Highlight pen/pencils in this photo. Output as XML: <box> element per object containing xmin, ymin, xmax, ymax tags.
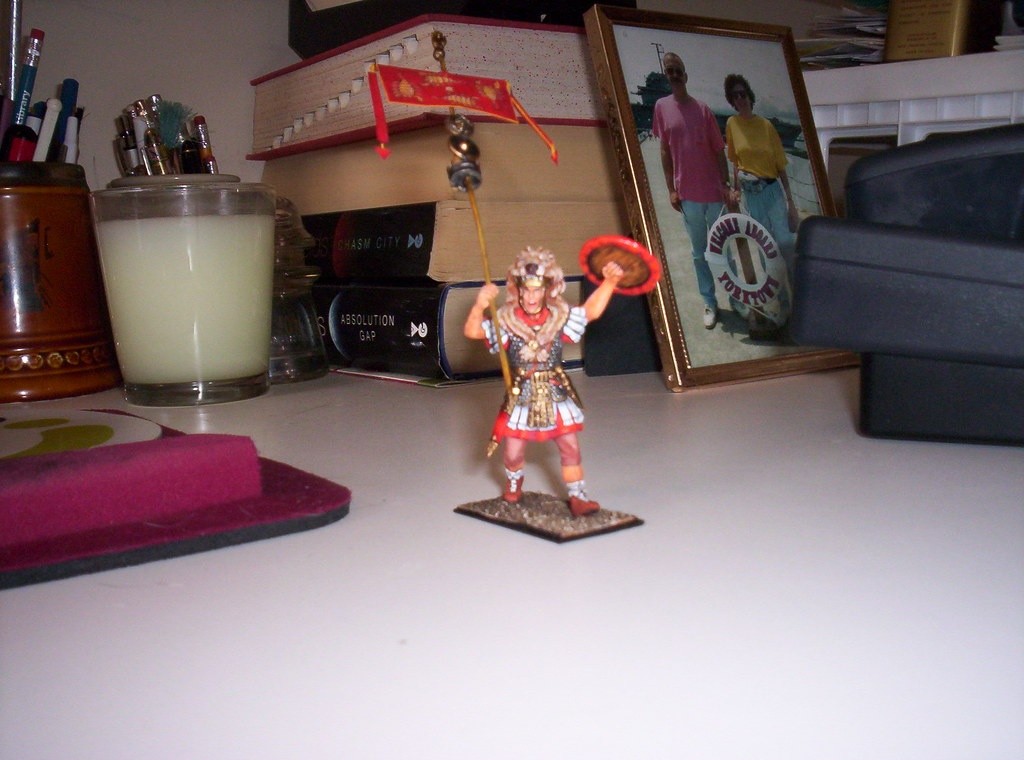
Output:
<box><xmin>115</xmin><ymin>94</ymin><xmax>219</xmax><ymax>176</ymax></box>
<box><xmin>12</xmin><ymin>27</ymin><xmax>44</xmax><ymax>123</ymax></box>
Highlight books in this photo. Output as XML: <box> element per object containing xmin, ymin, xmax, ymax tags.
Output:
<box><xmin>248</xmin><ymin>12</ymin><xmax>635</xmax><ymax>382</ymax></box>
<box><xmin>792</xmin><ymin>13</ymin><xmax>887</xmax><ymax>69</ymax></box>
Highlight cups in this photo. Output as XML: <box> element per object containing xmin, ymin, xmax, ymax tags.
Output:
<box><xmin>87</xmin><ymin>184</ymin><xmax>276</xmax><ymax>407</ymax></box>
<box><xmin>0</xmin><ymin>162</ymin><xmax>122</xmax><ymax>403</ymax></box>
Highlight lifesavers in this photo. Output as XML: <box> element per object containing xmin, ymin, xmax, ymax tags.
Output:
<box><xmin>703</xmin><ymin>211</ymin><xmax>787</xmax><ymax>308</ymax></box>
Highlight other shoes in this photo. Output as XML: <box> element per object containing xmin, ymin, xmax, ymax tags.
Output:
<box><xmin>703</xmin><ymin>295</ymin><xmax>719</xmax><ymax>329</ymax></box>
<box><xmin>731</xmin><ymin>299</ymin><xmax>751</xmax><ymax>320</ymax></box>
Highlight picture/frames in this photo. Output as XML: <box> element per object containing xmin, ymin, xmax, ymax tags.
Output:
<box><xmin>582</xmin><ymin>4</ymin><xmax>864</xmax><ymax>390</ymax></box>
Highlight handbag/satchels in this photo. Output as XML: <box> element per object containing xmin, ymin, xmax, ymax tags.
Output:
<box><xmin>738</xmin><ymin>170</ymin><xmax>768</xmax><ymax>194</ymax></box>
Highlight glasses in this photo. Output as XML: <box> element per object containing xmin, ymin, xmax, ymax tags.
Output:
<box><xmin>665</xmin><ymin>66</ymin><xmax>684</xmax><ymax>77</ymax></box>
<box><xmin>730</xmin><ymin>89</ymin><xmax>746</xmax><ymax>100</ymax></box>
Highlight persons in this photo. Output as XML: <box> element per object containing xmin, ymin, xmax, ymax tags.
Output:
<box><xmin>651</xmin><ymin>52</ymin><xmax>751</xmax><ymax>329</ymax></box>
<box><xmin>465</xmin><ymin>244</ymin><xmax>623</xmax><ymax>516</ymax></box>
<box><xmin>723</xmin><ymin>74</ymin><xmax>800</xmax><ymax>320</ymax></box>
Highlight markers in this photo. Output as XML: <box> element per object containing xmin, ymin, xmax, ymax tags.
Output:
<box><xmin>0</xmin><ymin>78</ymin><xmax>83</xmax><ymax>163</ymax></box>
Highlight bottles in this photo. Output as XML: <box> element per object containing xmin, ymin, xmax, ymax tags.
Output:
<box><xmin>268</xmin><ymin>196</ymin><xmax>329</xmax><ymax>383</ymax></box>
